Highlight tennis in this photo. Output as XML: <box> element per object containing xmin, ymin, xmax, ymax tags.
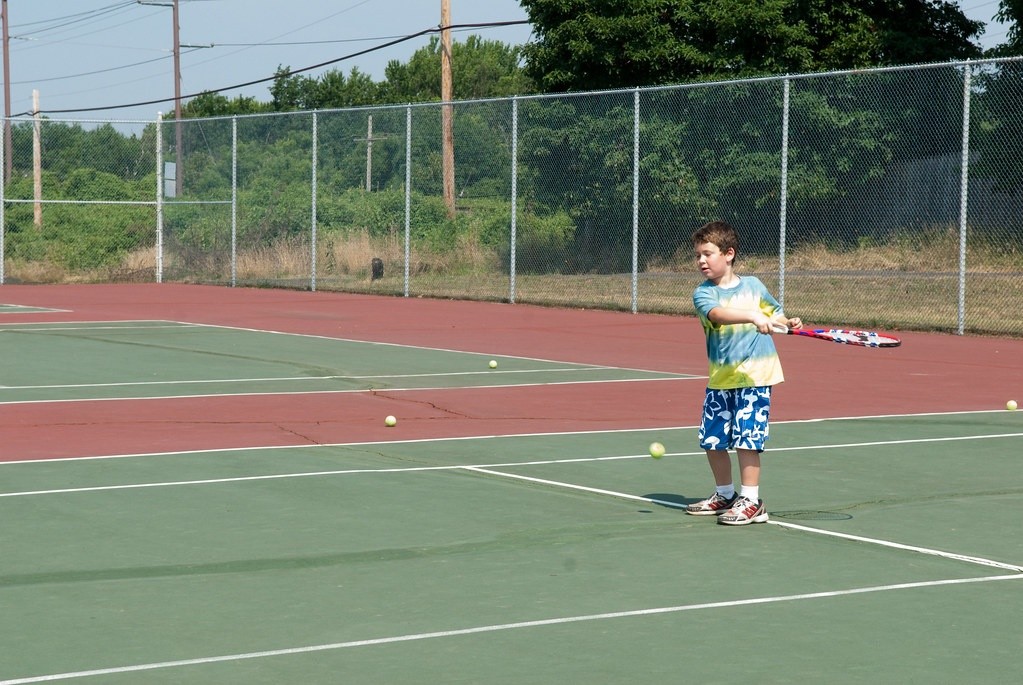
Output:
<box><xmin>649</xmin><ymin>441</ymin><xmax>665</xmax><ymax>458</ymax></box>
<box><xmin>385</xmin><ymin>415</ymin><xmax>397</xmax><ymax>427</ymax></box>
<box><xmin>1006</xmin><ymin>400</ymin><xmax>1019</xmax><ymax>411</ymax></box>
<box><xmin>489</xmin><ymin>359</ymin><xmax>498</xmax><ymax>369</ymax></box>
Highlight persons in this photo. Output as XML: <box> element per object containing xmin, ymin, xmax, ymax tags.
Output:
<box><xmin>686</xmin><ymin>222</ymin><xmax>803</xmax><ymax>526</ymax></box>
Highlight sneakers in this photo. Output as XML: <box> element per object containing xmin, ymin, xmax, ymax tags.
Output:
<box><xmin>716</xmin><ymin>497</ymin><xmax>769</xmax><ymax>524</ymax></box>
<box><xmin>687</xmin><ymin>490</ymin><xmax>739</xmax><ymax>515</ymax></box>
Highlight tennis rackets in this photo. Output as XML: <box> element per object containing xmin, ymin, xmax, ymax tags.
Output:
<box><xmin>755</xmin><ymin>321</ymin><xmax>902</xmax><ymax>348</ymax></box>
